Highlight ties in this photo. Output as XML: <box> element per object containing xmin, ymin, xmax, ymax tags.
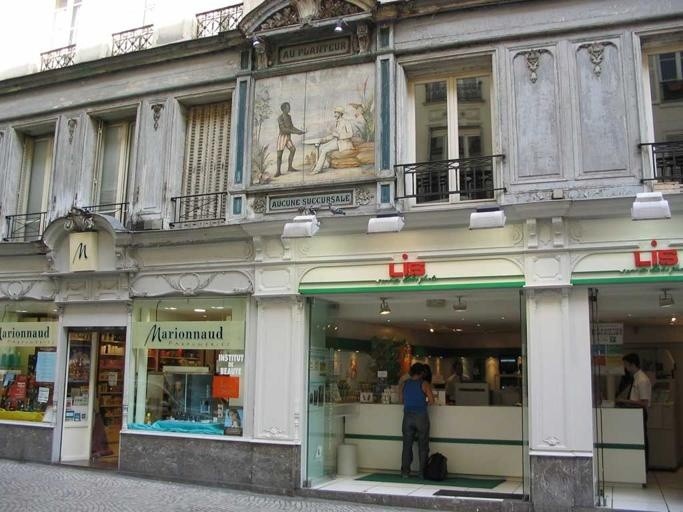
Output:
<box><xmin>626</xmin><ymin>376</ymin><xmax>634</xmax><ymax>400</ymax></box>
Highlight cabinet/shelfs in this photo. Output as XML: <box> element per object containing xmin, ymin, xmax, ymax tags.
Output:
<box><xmin>68</xmin><ymin>333</ymin><xmax>206</xmax><ymax>445</ymax></box>
<box><xmin>646</xmin><ymin>379</ymin><xmax>683</xmax><ymax>473</ymax></box>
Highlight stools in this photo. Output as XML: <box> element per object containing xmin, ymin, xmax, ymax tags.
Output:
<box><xmin>327</xmin><ymin>148</ymin><xmax>361</xmax><ymax>168</ymax></box>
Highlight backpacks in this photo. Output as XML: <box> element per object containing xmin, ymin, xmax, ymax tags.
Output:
<box><xmin>423</xmin><ymin>452</ymin><xmax>448</xmax><ymax>481</ymax></box>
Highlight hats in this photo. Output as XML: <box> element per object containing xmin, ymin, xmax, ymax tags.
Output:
<box><xmin>333</xmin><ymin>106</ymin><xmax>347</xmax><ymax>114</ymax></box>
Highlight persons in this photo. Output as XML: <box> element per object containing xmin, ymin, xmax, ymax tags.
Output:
<box><xmin>400</xmin><ymin>363</ymin><xmax>434</xmax><ymax>478</ymax></box>
<box><xmin>274</xmin><ymin>102</ymin><xmax>305</xmax><ymax>177</ymax></box>
<box><xmin>398</xmin><ymin>364</ymin><xmax>432</xmax><ymax>383</ymax></box>
<box><xmin>614</xmin><ymin>353</ymin><xmax>652</xmax><ymax>488</ymax></box>
<box><xmin>309</xmin><ymin>106</ymin><xmax>353</xmax><ymax>175</ymax></box>
<box><xmin>228</xmin><ymin>409</ymin><xmax>241</xmax><ymax>432</ymax></box>
<box><xmin>446</xmin><ymin>362</ymin><xmax>469</xmax><ymax>404</ymax></box>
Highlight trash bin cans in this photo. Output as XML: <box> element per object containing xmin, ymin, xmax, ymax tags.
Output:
<box><xmin>336</xmin><ymin>443</ymin><xmax>357</xmax><ymax>476</ymax></box>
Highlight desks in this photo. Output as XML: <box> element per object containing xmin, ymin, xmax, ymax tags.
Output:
<box><xmin>302</xmin><ymin>138</ymin><xmax>329</xmax><ymax>172</ymax></box>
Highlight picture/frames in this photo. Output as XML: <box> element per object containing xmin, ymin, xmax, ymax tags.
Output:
<box><xmin>33</xmin><ymin>346</ymin><xmax>57</xmax><ymax>386</ymax></box>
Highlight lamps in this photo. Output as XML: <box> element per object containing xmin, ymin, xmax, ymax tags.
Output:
<box><xmin>283</xmin><ymin>206</ymin><xmax>320</xmax><ymax>237</ymax></box>
<box><xmin>251</xmin><ymin>32</ymin><xmax>264</xmax><ymax>48</ymax></box>
<box><xmin>658</xmin><ymin>288</ymin><xmax>676</xmax><ymax>308</ymax></box>
<box><xmin>452</xmin><ymin>295</ymin><xmax>467</xmax><ymax>312</ymax></box>
<box><xmin>631</xmin><ymin>180</ymin><xmax>672</xmax><ymax>220</ymax></box>
<box><xmin>333</xmin><ymin>18</ymin><xmax>356</xmax><ymax>35</ymax></box>
<box><xmin>367</xmin><ymin>212</ymin><xmax>405</xmax><ymax>233</ymax></box>
<box><xmin>377</xmin><ymin>296</ymin><xmax>391</xmax><ymax>315</ymax></box>
<box><xmin>468</xmin><ymin>206</ymin><xmax>507</xmax><ymax>230</ymax></box>
<box><xmin>63</xmin><ymin>206</ymin><xmax>94</xmax><ymax>232</ymax></box>
